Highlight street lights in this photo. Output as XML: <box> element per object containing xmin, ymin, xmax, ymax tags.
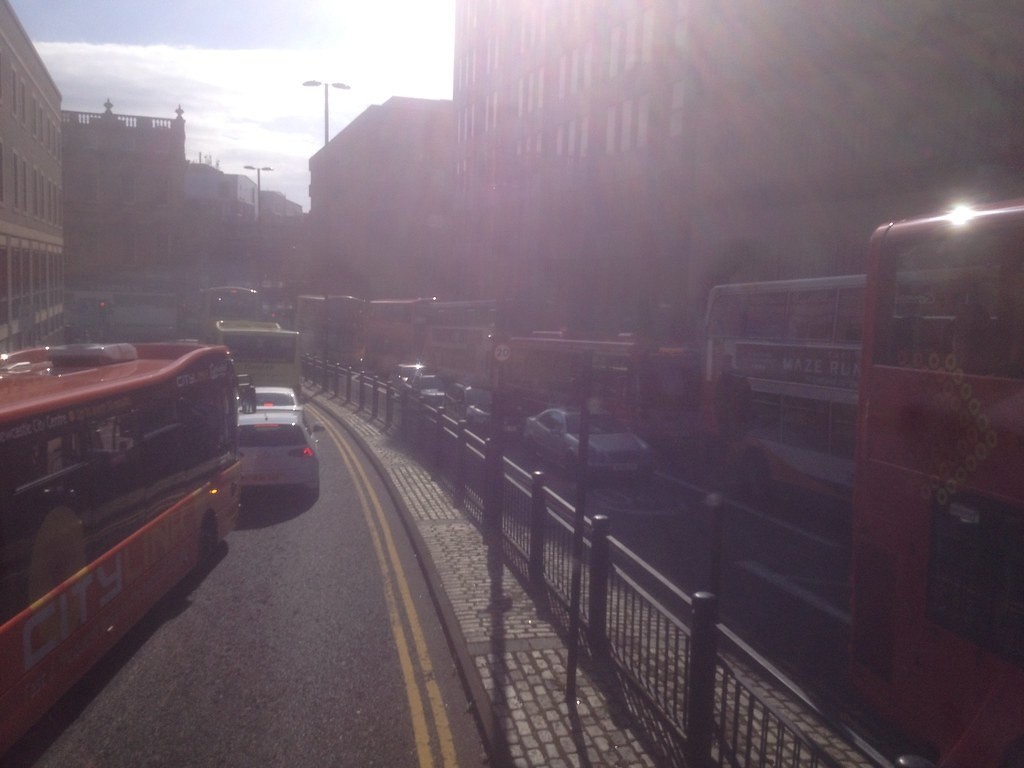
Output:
<box><xmin>244</xmin><ymin>164</ymin><xmax>275</xmax><ymax>220</ymax></box>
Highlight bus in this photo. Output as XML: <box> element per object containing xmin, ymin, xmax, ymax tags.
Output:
<box><xmin>705</xmin><ymin>272</ymin><xmax>941</xmax><ymax>412</ymax></box>
<box><xmin>216</xmin><ymin>318</ymin><xmax>302</xmax><ymax>397</ymax></box>
<box><xmin>0</xmin><ymin>338</ymin><xmax>258</xmax><ymax>755</ymax></box>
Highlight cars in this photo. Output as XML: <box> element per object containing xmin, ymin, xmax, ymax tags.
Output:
<box><xmin>525</xmin><ymin>406</ymin><xmax>654</xmax><ymax>479</ymax></box>
<box><xmin>236</xmin><ymin>386</ymin><xmax>313</xmax><ymax>439</ymax></box>
<box><xmin>236</xmin><ymin>410</ymin><xmax>326</xmax><ymax>497</ymax></box>
<box><xmin>393</xmin><ymin>356</ymin><xmax>531</xmax><ymax>443</ymax></box>
